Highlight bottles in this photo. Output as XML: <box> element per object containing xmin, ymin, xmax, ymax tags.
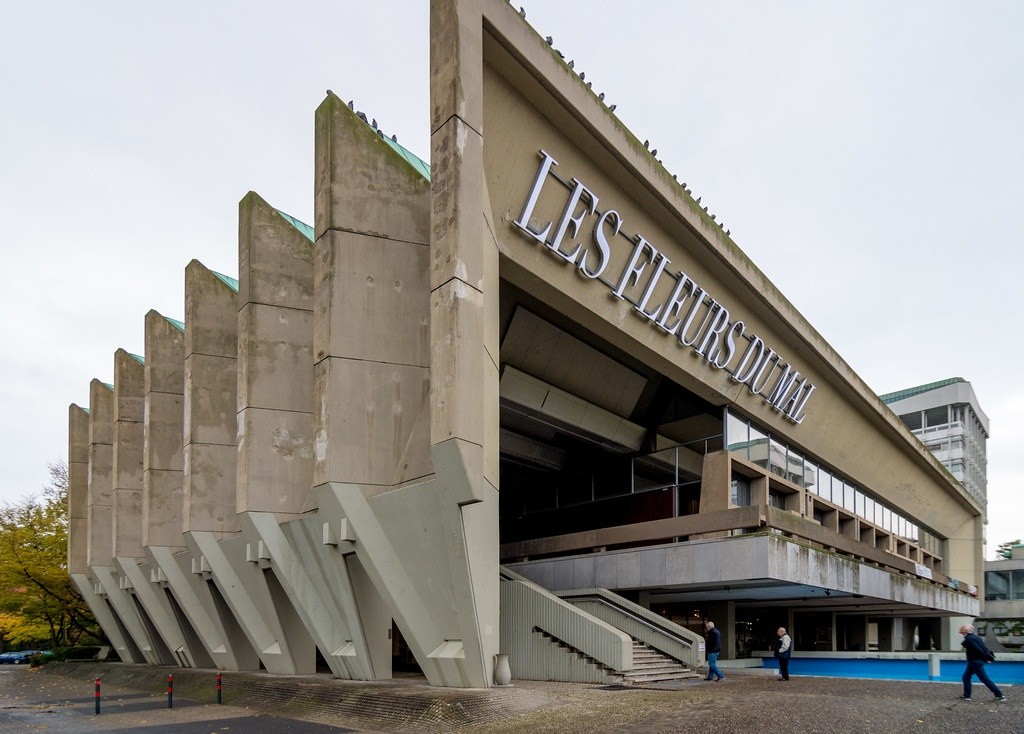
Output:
<box><xmin>495</xmin><ymin>654</ymin><xmax>511</xmax><ymax>685</ymax></box>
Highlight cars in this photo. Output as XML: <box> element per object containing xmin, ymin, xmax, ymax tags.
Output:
<box><xmin>0</xmin><ymin>649</ymin><xmax>44</xmax><ymax>665</ymax></box>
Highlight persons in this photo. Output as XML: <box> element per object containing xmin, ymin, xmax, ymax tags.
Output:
<box><xmin>959</xmin><ymin>625</ymin><xmax>1008</xmax><ymax>702</ymax></box>
<box><xmin>704</xmin><ymin>621</ymin><xmax>725</xmax><ymax>681</ymax></box>
<box><xmin>774</xmin><ymin>627</ymin><xmax>792</xmax><ymax>681</ymax></box>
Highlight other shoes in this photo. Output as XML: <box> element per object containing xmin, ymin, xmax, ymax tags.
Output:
<box><xmin>778</xmin><ymin>678</ymin><xmax>788</xmax><ymax>681</ymax></box>
<box><xmin>959</xmin><ymin>696</ymin><xmax>972</xmax><ymax>701</ymax></box>
<box><xmin>715</xmin><ymin>677</ymin><xmax>725</xmax><ymax>682</ymax></box>
<box><xmin>704</xmin><ymin>677</ymin><xmax>713</xmax><ymax>682</ymax></box>
<box><xmin>993</xmin><ymin>695</ymin><xmax>1007</xmax><ymax>703</ymax></box>
